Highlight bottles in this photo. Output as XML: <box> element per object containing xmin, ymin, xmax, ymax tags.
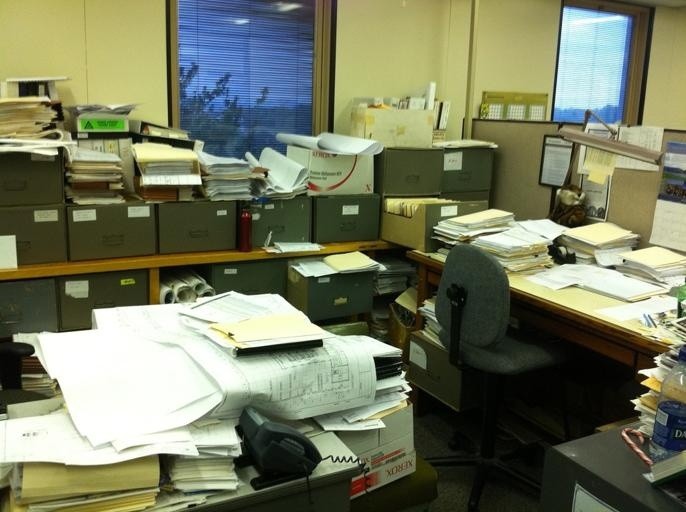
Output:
<box><xmin>648</xmin><ymin>345</ymin><xmax>686</xmax><ymax>465</ymax></box>
<box><xmin>237</xmin><ymin>205</ymin><xmax>253</xmax><ymax>253</ymax></box>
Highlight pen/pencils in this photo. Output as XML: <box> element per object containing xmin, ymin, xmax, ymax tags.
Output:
<box><xmin>644</xmin><ymin>313</ymin><xmax>657</xmax><ymax>328</ymax></box>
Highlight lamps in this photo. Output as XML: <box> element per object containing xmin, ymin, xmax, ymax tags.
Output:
<box><xmin>548</xmin><ymin>107</ymin><xmax>669</xmax><ymax>229</ymax></box>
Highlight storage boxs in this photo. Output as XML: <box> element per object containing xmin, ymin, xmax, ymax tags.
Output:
<box><xmin>0</xmin><ymin>138</ymin><xmax>495</xmax><ymax>414</ymax></box>
<box><xmin>348</xmin><ymin>95</ymin><xmax>435</xmax><ymax>145</ymax></box>
<box><xmin>538</xmin><ymin>420</ymin><xmax>685</xmax><ymax>511</ymax></box>
<box><xmin>336</xmin><ymin>400</ymin><xmax>419</xmax><ymax>503</ymax></box>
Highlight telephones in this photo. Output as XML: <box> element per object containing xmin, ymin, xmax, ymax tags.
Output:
<box><xmin>235</xmin><ymin>405</ymin><xmax>322</xmax><ymax>475</ymax></box>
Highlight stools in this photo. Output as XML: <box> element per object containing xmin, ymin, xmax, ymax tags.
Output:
<box><xmin>353</xmin><ymin>450</ymin><xmax>439</xmax><ymax>511</ymax></box>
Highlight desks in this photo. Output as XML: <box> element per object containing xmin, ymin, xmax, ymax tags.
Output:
<box><xmin>169</xmin><ymin>430</ymin><xmax>361</xmax><ymax>511</ymax></box>
<box><xmin>402</xmin><ymin>248</ymin><xmax>673</xmax><ymax>415</ymax></box>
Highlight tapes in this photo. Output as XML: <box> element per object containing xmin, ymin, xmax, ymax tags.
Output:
<box><xmin>558</xmin><ymin>246</ymin><xmax>568</xmax><ymax>256</ymax></box>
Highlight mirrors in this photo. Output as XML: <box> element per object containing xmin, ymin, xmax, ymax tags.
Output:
<box><xmin>463</xmin><ymin>0</ymin><xmax>685</xmax><ymax>207</ymax></box>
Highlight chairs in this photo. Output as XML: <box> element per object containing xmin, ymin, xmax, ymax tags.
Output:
<box><xmin>435</xmin><ymin>242</ymin><xmax>581</xmax><ymax>512</ymax></box>
